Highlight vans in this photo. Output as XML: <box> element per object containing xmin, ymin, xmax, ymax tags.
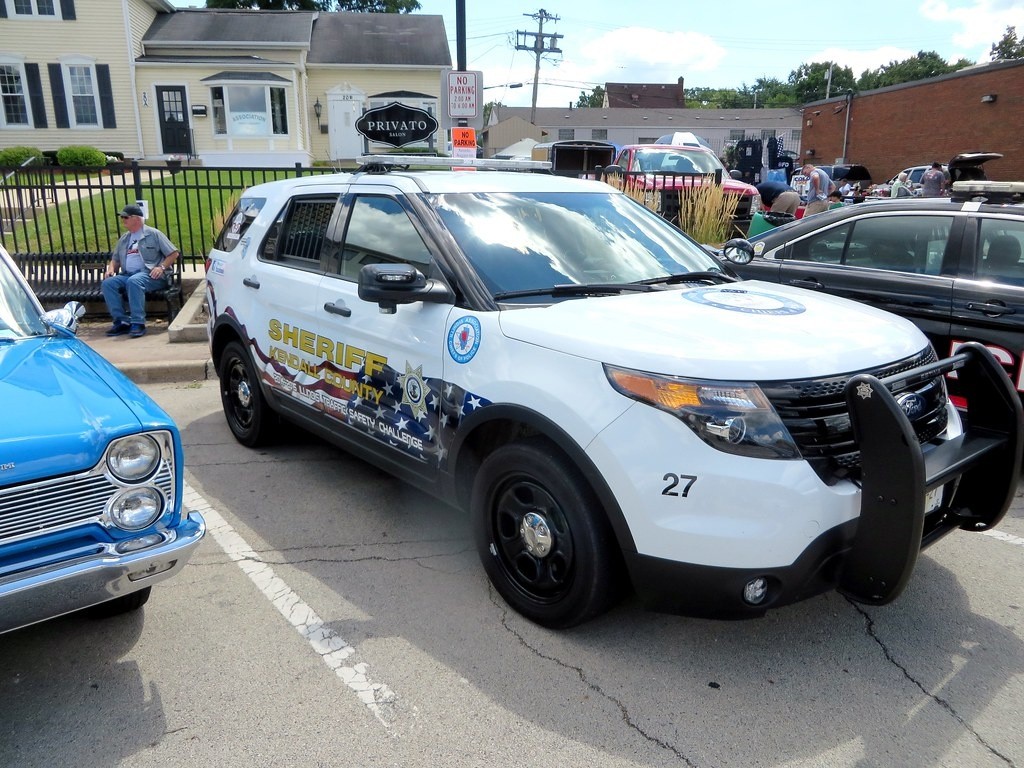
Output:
<box><xmin>791</xmin><ymin>164</ymin><xmax>871</xmax><ymax>195</ymax></box>
<box><xmin>883</xmin><ymin>152</ymin><xmax>1004</xmax><ymax>200</ymax></box>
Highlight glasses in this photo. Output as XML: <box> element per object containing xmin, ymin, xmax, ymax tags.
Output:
<box><xmin>121</xmin><ymin>216</ymin><xmax>134</xmax><ymax>219</ymax></box>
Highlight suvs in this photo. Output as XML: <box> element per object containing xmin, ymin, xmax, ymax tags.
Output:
<box><xmin>205</xmin><ymin>153</ymin><xmax>1024</xmax><ymax>631</ymax></box>
<box><xmin>607</xmin><ymin>144</ymin><xmax>761</xmax><ymax>241</ymax></box>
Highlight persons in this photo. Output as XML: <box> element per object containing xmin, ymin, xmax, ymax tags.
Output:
<box><xmin>756</xmin><ymin>181</ymin><xmax>800</xmax><ymax>217</ymax></box>
<box><xmin>100</xmin><ymin>205</ymin><xmax>180</xmax><ymax>338</ymax></box>
<box><xmin>891</xmin><ymin>173</ymin><xmax>915</xmax><ymax>199</ymax></box>
<box><xmin>829</xmin><ymin>190</ymin><xmax>845</xmax><ymax>209</ymax></box>
<box><xmin>919</xmin><ymin>161</ymin><xmax>946</xmax><ymax>197</ymax></box>
<box><xmin>838</xmin><ymin>179</ymin><xmax>852</xmax><ymax>195</ymax></box>
<box><xmin>801</xmin><ymin>163</ymin><xmax>836</xmax><ymax>219</ymax></box>
<box><xmin>938</xmin><ymin>164</ymin><xmax>951</xmax><ymax>185</ymax></box>
<box><xmin>848</xmin><ymin>182</ymin><xmax>862</xmax><ymax>197</ymax></box>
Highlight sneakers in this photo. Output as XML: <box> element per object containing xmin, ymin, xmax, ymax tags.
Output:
<box><xmin>105</xmin><ymin>324</ymin><xmax>132</xmax><ymax>335</ymax></box>
<box><xmin>129</xmin><ymin>323</ymin><xmax>146</xmax><ymax>336</ymax></box>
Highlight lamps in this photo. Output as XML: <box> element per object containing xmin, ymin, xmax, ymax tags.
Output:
<box><xmin>980</xmin><ymin>94</ymin><xmax>997</xmax><ymax>102</ymax></box>
<box><xmin>314</xmin><ymin>97</ymin><xmax>322</xmax><ymax>129</ymax></box>
<box><xmin>806</xmin><ymin>149</ymin><xmax>816</xmax><ymax>155</ymax></box>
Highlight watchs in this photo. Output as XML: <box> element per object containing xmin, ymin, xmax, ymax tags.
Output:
<box><xmin>159</xmin><ymin>263</ymin><xmax>166</xmax><ymax>270</ymax></box>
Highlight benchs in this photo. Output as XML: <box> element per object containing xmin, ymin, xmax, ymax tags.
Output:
<box><xmin>10</xmin><ymin>250</ymin><xmax>185</xmax><ymax>328</ymax></box>
<box><xmin>833</xmin><ymin>240</ymin><xmax>947</xmax><ymax>274</ymax></box>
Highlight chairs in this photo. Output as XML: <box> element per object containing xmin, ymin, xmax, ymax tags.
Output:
<box><xmin>985</xmin><ymin>236</ymin><xmax>1024</xmax><ymax>289</ymax></box>
<box><xmin>676</xmin><ymin>157</ymin><xmax>696</xmax><ymax>173</ymax></box>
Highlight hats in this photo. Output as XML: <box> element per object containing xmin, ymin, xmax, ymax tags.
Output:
<box><xmin>115</xmin><ymin>205</ymin><xmax>143</xmax><ymax>217</ymax></box>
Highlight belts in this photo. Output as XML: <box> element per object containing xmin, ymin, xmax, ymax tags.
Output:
<box><xmin>782</xmin><ymin>190</ymin><xmax>797</xmax><ymax>192</ymax></box>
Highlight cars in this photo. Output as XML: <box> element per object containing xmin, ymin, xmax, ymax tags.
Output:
<box><xmin>0</xmin><ymin>244</ymin><xmax>205</xmax><ymax>638</ymax></box>
<box><xmin>705</xmin><ymin>180</ymin><xmax>1024</xmax><ymax>434</ymax></box>
